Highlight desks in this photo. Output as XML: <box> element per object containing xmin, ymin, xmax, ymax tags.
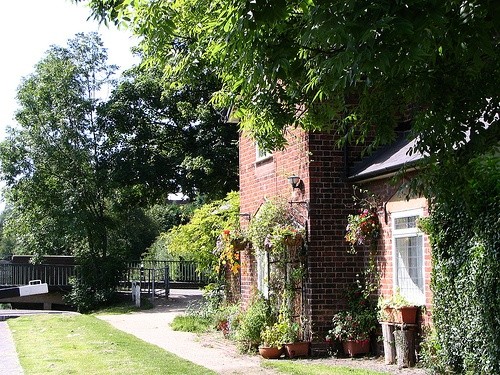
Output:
<box><xmin>379</xmin><ymin>322</ymin><xmax>420</xmax><ymax>369</ymax></box>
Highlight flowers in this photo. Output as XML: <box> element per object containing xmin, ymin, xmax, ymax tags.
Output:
<box><xmin>324</xmin><ymin>285</ymin><xmax>378</xmax><ymax>356</ymax></box>
<box><xmin>342</xmin><ymin>183</ymin><xmax>380</xmax><ymax>254</ymax></box>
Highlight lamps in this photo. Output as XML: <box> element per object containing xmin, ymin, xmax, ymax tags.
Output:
<box><xmin>288</xmin><ymin>173</ymin><xmax>301</xmax><ymax>189</ymax></box>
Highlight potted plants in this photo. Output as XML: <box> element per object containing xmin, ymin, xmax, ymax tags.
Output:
<box><xmin>376</xmin><ymin>290</ymin><xmax>417</xmax><ymax>324</ymax></box>
<box><xmin>214</xmin><ymin>199</ymin><xmax>309</xmax><ymax>358</ymax></box>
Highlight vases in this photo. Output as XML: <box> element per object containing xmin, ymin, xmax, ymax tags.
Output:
<box><xmin>342</xmin><ymin>339</ymin><xmax>369</xmax><ymax>355</ymax></box>
